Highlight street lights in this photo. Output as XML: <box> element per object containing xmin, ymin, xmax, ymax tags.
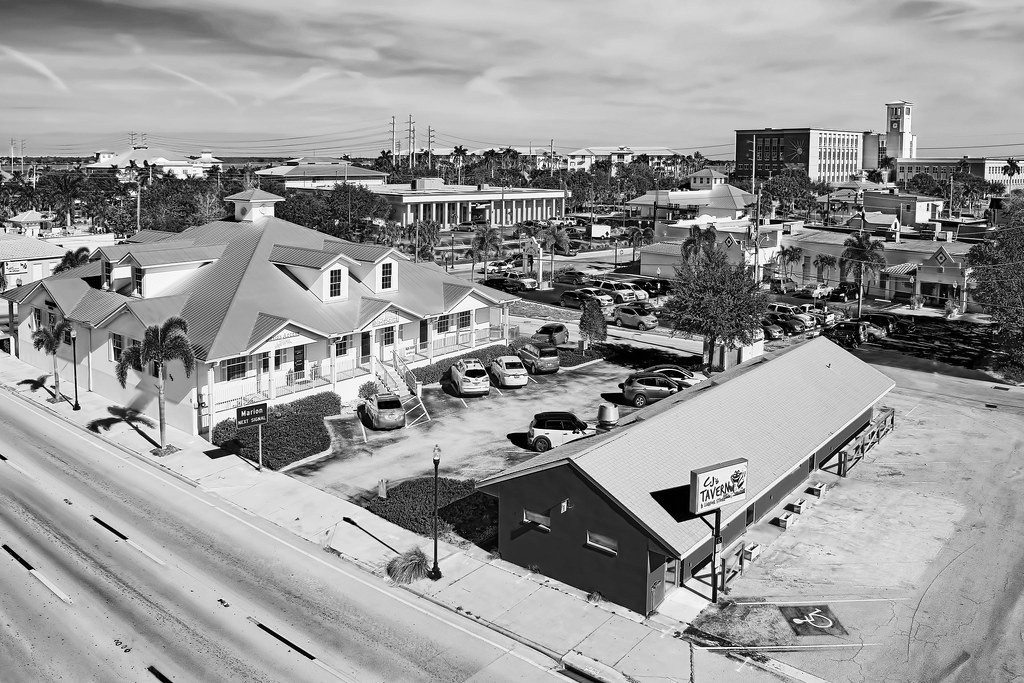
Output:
<box><xmin>429</xmin><ymin>444</ymin><xmax>442</xmax><ymax>580</ymax></box>
<box><xmin>70</xmin><ymin>329</ymin><xmax>81</xmax><ymax>410</ymax></box>
<box><xmin>754</xmin><ymin>234</ymin><xmax>770</xmax><ymax>284</ymax></box>
<box><xmin>656</xmin><ymin>267</ymin><xmax>661</xmax><ymax>304</ymax></box>
<box><xmin>909</xmin><ymin>276</ymin><xmax>914</xmax><ymax>309</ymax></box>
<box><xmin>614</xmin><ymin>239</ymin><xmax>618</xmax><ymax>269</ymax></box>
<box><xmin>451</xmin><ymin>233</ymin><xmax>454</xmax><ymax>269</ymax></box>
<box><xmin>953</xmin><ymin>280</ymin><xmax>958</xmax><ymax>304</ymax></box>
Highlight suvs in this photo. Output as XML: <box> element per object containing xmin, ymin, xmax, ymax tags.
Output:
<box><xmin>770</xmin><ymin>277</ymin><xmax>798</xmax><ymax>295</ymax></box>
<box><xmin>830</xmin><ymin>281</ymin><xmax>860</xmax><ymax>302</ymax></box>
<box><xmin>623</xmin><ymin>371</ymin><xmax>692</xmax><ymax>408</ymax></box>
<box><xmin>450</xmin><ymin>357</ymin><xmax>491</xmax><ymax>396</ymax></box>
<box><xmin>451</xmin><ymin>220</ymin><xmax>490</xmax><ymax>232</ymax></box>
<box><xmin>518</xmin><ymin>343</ymin><xmax>560</xmax><ymax>375</ymax></box>
<box><xmin>526</xmin><ymin>411</ymin><xmax>596</xmax><ymax>453</ymax></box>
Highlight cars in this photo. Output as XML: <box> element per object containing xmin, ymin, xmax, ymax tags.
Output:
<box><xmin>365</xmin><ymin>393</ymin><xmax>405</xmax><ymax>430</ymax></box>
<box><xmin>479</xmin><ymin>253</ymin><xmax>538</xmax><ymax>293</ymax></box>
<box><xmin>513</xmin><ymin>216</ymin><xmax>611</xmax><ymax>256</ymax></box>
<box><xmin>561</xmin><ymin>279</ymin><xmax>679</xmax><ymax>331</ymax></box>
<box><xmin>750</xmin><ymin>302</ymin><xmax>916</xmax><ymax>350</ymax></box>
<box><xmin>801</xmin><ymin>282</ymin><xmax>835</xmax><ymax>300</ymax></box>
<box><xmin>636</xmin><ymin>364</ymin><xmax>709</xmax><ymax>386</ymax></box>
<box><xmin>491</xmin><ymin>355</ymin><xmax>529</xmax><ymax>388</ymax></box>
<box><xmin>531</xmin><ymin>323</ymin><xmax>569</xmax><ymax>345</ymax></box>
<box><xmin>554</xmin><ymin>271</ymin><xmax>590</xmax><ymax>286</ymax></box>
